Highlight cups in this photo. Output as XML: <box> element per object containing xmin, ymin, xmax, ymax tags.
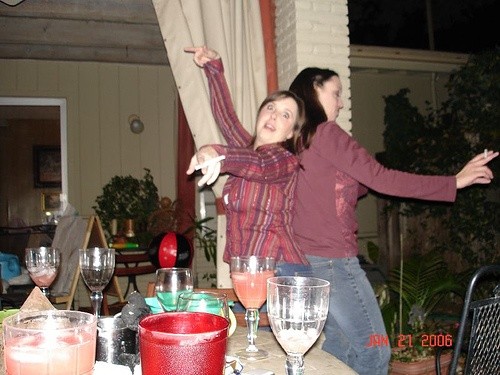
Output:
<box><xmin>178</xmin><ymin>292</ymin><xmax>229</xmax><ymax>320</ymax></box>
<box><xmin>95</xmin><ymin>317</ymin><xmax>136</xmax><ymax>374</ymax></box>
<box><xmin>155</xmin><ymin>267</ymin><xmax>193</xmax><ymax>312</ymax></box>
<box><xmin>2</xmin><ymin>309</ymin><xmax>97</xmax><ymax>375</ymax></box>
<box><xmin>138</xmin><ymin>311</ymin><xmax>229</xmax><ymax>375</ymax></box>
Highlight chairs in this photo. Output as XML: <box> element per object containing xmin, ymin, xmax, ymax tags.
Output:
<box><xmin>435</xmin><ymin>262</ymin><xmax>500</xmax><ymax>375</ymax></box>
<box><xmin>114</xmin><ymin>250</ymin><xmax>160</xmax><ymax>278</ymax></box>
<box><xmin>145</xmin><ymin>281</ymin><xmax>271</xmax><ymax>327</ymax></box>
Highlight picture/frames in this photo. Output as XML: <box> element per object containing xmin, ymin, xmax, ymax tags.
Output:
<box><xmin>32</xmin><ymin>144</ymin><xmax>61</xmax><ymax>189</ymax></box>
<box><xmin>40</xmin><ymin>191</ymin><xmax>61</xmax><ymax>212</ymax></box>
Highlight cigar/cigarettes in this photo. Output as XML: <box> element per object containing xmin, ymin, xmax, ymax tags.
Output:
<box><xmin>483</xmin><ymin>147</ymin><xmax>487</xmax><ymax>160</ymax></box>
<box><xmin>195</xmin><ymin>154</ymin><xmax>226</xmax><ymax>171</ymax></box>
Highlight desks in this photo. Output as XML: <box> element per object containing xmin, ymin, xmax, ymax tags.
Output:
<box><xmin>224</xmin><ymin>324</ymin><xmax>360</xmax><ymax>375</ymax></box>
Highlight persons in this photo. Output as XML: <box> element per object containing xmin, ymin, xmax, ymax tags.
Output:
<box><xmin>185</xmin><ymin>45</ymin><xmax>314</xmax><ymax>311</ymax></box>
<box><xmin>287</xmin><ymin>66</ymin><xmax>499</xmax><ymax>375</ymax></box>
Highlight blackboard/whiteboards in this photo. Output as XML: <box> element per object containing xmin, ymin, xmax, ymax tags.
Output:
<box><xmin>45</xmin><ymin>214</ymin><xmax>121</xmax><ymax>303</ymax></box>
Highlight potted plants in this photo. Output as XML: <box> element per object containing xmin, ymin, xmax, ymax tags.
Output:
<box><xmin>355</xmin><ymin>234</ymin><xmax>486</xmax><ymax>375</ymax></box>
<box><xmin>89</xmin><ymin>166</ymin><xmax>161</xmax><ymax>245</ymax></box>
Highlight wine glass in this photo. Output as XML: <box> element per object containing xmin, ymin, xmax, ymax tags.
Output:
<box><xmin>267</xmin><ymin>276</ymin><xmax>330</xmax><ymax>375</ymax></box>
<box><xmin>24</xmin><ymin>248</ymin><xmax>60</xmax><ymax>299</ymax></box>
<box><xmin>230</xmin><ymin>256</ymin><xmax>277</xmax><ymax>360</ymax></box>
<box><xmin>78</xmin><ymin>248</ymin><xmax>116</xmax><ymax>318</ymax></box>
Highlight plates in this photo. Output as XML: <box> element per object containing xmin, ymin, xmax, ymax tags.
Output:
<box><xmin>224</xmin><ymin>355</ymin><xmax>243</xmax><ymax>375</ymax></box>
<box><xmin>146</xmin><ymin>296</ymin><xmax>238</xmax><ymax>338</ymax></box>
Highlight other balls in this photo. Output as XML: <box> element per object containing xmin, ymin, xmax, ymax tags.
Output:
<box><xmin>147</xmin><ymin>231</ymin><xmax>193</xmax><ymax>273</ymax></box>
